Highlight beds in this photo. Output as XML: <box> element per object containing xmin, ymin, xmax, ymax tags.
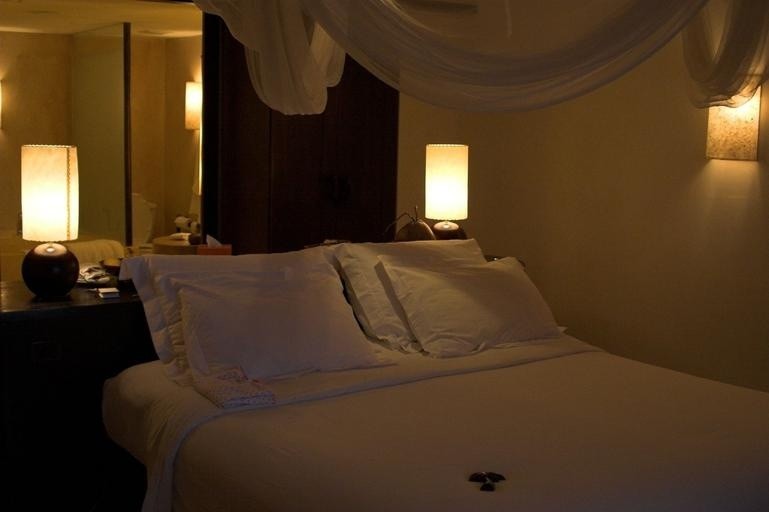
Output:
<box><xmin>101</xmin><ymin>335</ymin><xmax>768</xmax><ymax>512</ymax></box>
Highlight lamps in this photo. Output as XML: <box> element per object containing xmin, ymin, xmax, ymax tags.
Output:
<box><xmin>22</xmin><ymin>144</ymin><xmax>80</xmax><ymax>302</ymax></box>
<box><xmin>424</xmin><ymin>144</ymin><xmax>469</xmax><ymax>240</ymax></box>
<box><xmin>705</xmin><ymin>74</ymin><xmax>763</xmax><ymax>162</ymax></box>
<box><xmin>185</xmin><ymin>82</ymin><xmax>200</xmax><ymax>130</ymax></box>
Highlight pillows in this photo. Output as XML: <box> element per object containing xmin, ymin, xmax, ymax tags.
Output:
<box><xmin>329</xmin><ymin>236</ymin><xmax>487</xmax><ymax>352</ymax></box>
<box><xmin>379</xmin><ymin>252</ymin><xmax>568</xmax><ymax>358</ymax></box>
<box><xmin>156</xmin><ymin>264</ymin><xmax>399</xmax><ymax>382</ymax></box>
<box><xmin>117</xmin><ymin>244</ymin><xmax>343</xmax><ymax>387</ymax></box>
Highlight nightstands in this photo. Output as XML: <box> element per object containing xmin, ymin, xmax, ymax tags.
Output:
<box><xmin>0</xmin><ymin>274</ymin><xmax>139</xmax><ymax>326</ymax></box>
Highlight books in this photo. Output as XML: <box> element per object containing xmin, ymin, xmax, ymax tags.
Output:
<box><xmin>98</xmin><ymin>287</ymin><xmax>119</xmax><ymax>299</ymax></box>
